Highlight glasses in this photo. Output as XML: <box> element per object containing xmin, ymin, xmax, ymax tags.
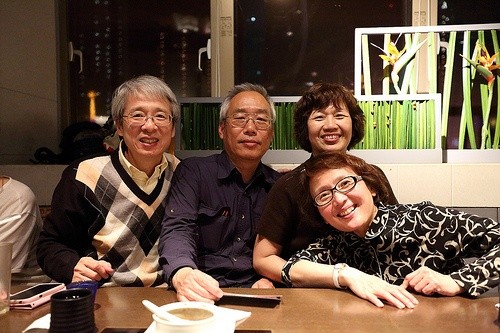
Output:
<box><xmin>226</xmin><ymin>112</ymin><xmax>274</xmax><ymax>130</ymax></box>
<box><xmin>313</xmin><ymin>175</ymin><xmax>362</xmax><ymax>206</ymax></box>
<box><xmin>121</xmin><ymin>111</ymin><xmax>176</xmax><ymax>127</ymax></box>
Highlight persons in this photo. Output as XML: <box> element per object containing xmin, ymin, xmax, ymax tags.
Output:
<box><xmin>281</xmin><ymin>153</ymin><xmax>500</xmax><ymax>309</ymax></box>
<box><xmin>36</xmin><ymin>76</ymin><xmax>183</xmax><ymax>287</ymax></box>
<box><xmin>158</xmin><ymin>83</ymin><xmax>281</xmax><ymax>306</ymax></box>
<box><xmin>0</xmin><ymin>174</ymin><xmax>57</xmax><ymax>283</ymax></box>
<box><xmin>253</xmin><ymin>82</ymin><xmax>398</xmax><ymax>282</ymax></box>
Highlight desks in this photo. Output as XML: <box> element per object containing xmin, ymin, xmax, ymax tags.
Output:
<box><xmin>0</xmin><ymin>279</ymin><xmax>500</xmax><ymax>333</ymax></box>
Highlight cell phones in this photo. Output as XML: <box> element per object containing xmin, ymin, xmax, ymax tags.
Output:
<box><xmin>215</xmin><ymin>295</ymin><xmax>281</xmax><ymax>308</ymax></box>
<box><xmin>7</xmin><ymin>282</ymin><xmax>66</xmax><ymax>310</ymax></box>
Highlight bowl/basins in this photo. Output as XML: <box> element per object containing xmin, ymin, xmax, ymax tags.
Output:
<box><xmin>152</xmin><ymin>300</ymin><xmax>222</xmax><ymax>333</ymax></box>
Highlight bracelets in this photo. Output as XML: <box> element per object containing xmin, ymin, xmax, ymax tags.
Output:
<box><xmin>332</xmin><ymin>263</ymin><xmax>349</xmax><ymax>290</ymax></box>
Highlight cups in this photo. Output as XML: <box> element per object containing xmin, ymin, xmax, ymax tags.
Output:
<box><xmin>0</xmin><ymin>242</ymin><xmax>13</xmax><ymax>314</ymax></box>
<box><xmin>48</xmin><ymin>288</ymin><xmax>98</xmax><ymax>333</ymax></box>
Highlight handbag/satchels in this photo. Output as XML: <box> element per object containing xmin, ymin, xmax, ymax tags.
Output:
<box><xmin>29</xmin><ymin>121</ymin><xmax>106</xmax><ymax>165</ymax></box>
